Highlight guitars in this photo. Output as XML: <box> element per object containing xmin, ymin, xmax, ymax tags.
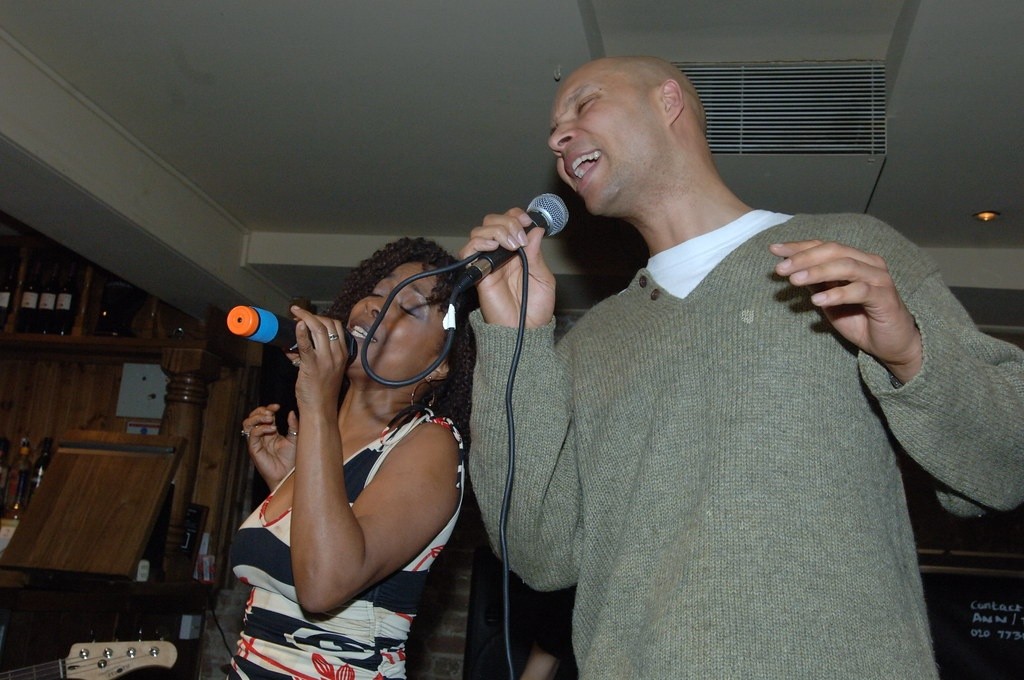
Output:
<box><xmin>0</xmin><ymin>629</ymin><xmax>178</xmax><ymax>680</ymax></box>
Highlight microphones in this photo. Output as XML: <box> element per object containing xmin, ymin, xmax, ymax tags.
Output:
<box><xmin>457</xmin><ymin>191</ymin><xmax>569</xmax><ymax>292</ymax></box>
<box><xmin>226</xmin><ymin>305</ymin><xmax>359</xmax><ymax>367</ymax></box>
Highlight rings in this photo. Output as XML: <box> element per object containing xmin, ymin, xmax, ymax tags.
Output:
<box><xmin>241</xmin><ymin>430</ymin><xmax>251</xmax><ymax>437</ymax></box>
<box><xmin>287</xmin><ymin>430</ymin><xmax>298</xmax><ymax>436</ymax></box>
<box><xmin>329</xmin><ymin>333</ymin><xmax>339</xmax><ymax>342</ymax></box>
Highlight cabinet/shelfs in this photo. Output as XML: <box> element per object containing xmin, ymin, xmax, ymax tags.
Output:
<box><xmin>0</xmin><ymin>212</ymin><xmax>203</xmax><ymax>345</ymax></box>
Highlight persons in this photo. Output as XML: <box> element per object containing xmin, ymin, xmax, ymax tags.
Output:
<box><xmin>221</xmin><ymin>236</ymin><xmax>478</xmax><ymax>680</ymax></box>
<box><xmin>458</xmin><ymin>55</ymin><xmax>1024</xmax><ymax>680</ymax></box>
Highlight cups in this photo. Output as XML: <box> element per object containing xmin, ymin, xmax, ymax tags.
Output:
<box><xmin>94</xmin><ymin>273</ymin><xmax>144</xmax><ymax>336</ymax></box>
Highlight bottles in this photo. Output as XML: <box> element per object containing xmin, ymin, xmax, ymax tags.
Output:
<box><xmin>16</xmin><ymin>261</ymin><xmax>42</xmax><ymax>333</ymax></box>
<box><xmin>48</xmin><ymin>261</ymin><xmax>78</xmax><ymax>334</ymax></box>
<box><xmin>0</xmin><ymin>262</ymin><xmax>16</xmax><ymax>333</ymax></box>
<box><xmin>0</xmin><ymin>436</ymin><xmax>10</xmax><ymax>516</ymax></box>
<box><xmin>32</xmin><ymin>262</ymin><xmax>60</xmax><ymax>332</ymax></box>
<box><xmin>22</xmin><ymin>436</ymin><xmax>53</xmax><ymax>516</ymax></box>
<box><xmin>4</xmin><ymin>438</ymin><xmax>34</xmax><ymax>519</ymax></box>
